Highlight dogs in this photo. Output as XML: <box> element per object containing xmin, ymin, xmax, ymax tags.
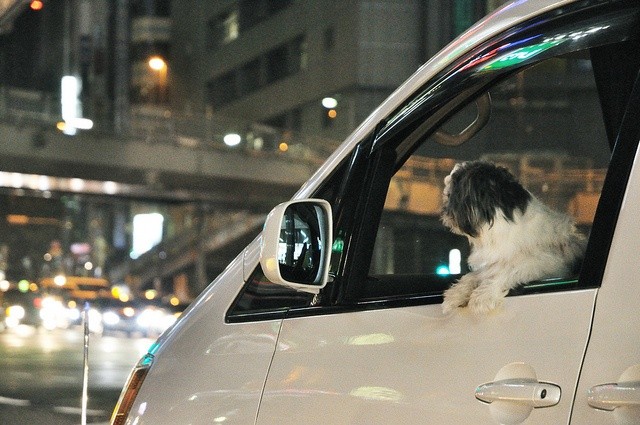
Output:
<box><xmin>439</xmin><ymin>159</ymin><xmax>589</xmax><ymax>322</ymax></box>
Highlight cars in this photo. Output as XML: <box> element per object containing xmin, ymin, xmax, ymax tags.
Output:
<box><xmin>0</xmin><ymin>276</ymin><xmax>191</xmax><ymax>338</ymax></box>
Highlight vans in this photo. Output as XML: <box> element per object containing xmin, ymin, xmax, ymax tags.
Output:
<box><xmin>105</xmin><ymin>0</ymin><xmax>640</xmax><ymax>425</ymax></box>
